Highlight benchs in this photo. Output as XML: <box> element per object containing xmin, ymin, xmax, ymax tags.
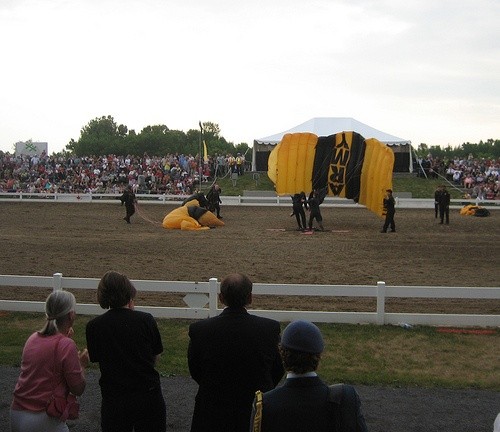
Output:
<box><xmin>242</xmin><ymin>189</ymin><xmax>277</xmax><ymax>203</ymax></box>
<box><xmin>279</xmin><ymin>194</ymin><xmax>309</xmax><ymax>203</ymax></box>
<box><xmin>392</xmin><ymin>191</ymin><xmax>413</xmax><ymax>198</ymax></box>
<box><xmin>326</xmin><ymin>195</ymin><xmax>356</xmax><ymax>204</ymax></box>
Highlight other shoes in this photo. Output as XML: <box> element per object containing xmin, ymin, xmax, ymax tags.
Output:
<box><xmin>123</xmin><ymin>217</ymin><xmax>131</xmax><ymax>224</ymax></box>
<box><xmin>380</xmin><ymin>231</ymin><xmax>387</xmax><ymax>233</ymax></box>
<box><xmin>217</xmin><ymin>216</ymin><xmax>223</xmax><ymax>219</ymax></box>
<box><xmin>438</xmin><ymin>222</ymin><xmax>449</xmax><ymax>225</ymax></box>
<box><xmin>389</xmin><ymin>230</ymin><xmax>395</xmax><ymax>232</ymax></box>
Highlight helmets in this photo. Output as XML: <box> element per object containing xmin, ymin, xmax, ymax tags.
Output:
<box><xmin>280</xmin><ymin>319</ymin><xmax>325</xmax><ymax>354</ymax></box>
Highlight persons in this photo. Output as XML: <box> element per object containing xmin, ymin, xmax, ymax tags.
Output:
<box><xmin>86</xmin><ymin>271</ymin><xmax>167</xmax><ymax>432</ymax></box>
<box><xmin>0</xmin><ymin>147</ymin><xmax>245</xmax><ymax>230</ymax></box>
<box><xmin>418</xmin><ymin>152</ymin><xmax>500</xmax><ymax>225</ymax></box>
<box><xmin>188</xmin><ymin>273</ymin><xmax>284</xmax><ymax>432</ymax></box>
<box><xmin>250</xmin><ymin>321</ymin><xmax>364</xmax><ymax>432</ymax></box>
<box><xmin>379</xmin><ymin>190</ymin><xmax>396</xmax><ymax>233</ymax></box>
<box><xmin>7</xmin><ymin>290</ymin><xmax>89</xmax><ymax>432</ymax></box>
<box><xmin>286</xmin><ymin>188</ymin><xmax>324</xmax><ymax>232</ymax></box>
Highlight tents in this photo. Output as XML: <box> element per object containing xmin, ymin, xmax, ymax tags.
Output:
<box><xmin>252</xmin><ymin>116</ymin><xmax>417</xmax><ymax>173</ymax></box>
